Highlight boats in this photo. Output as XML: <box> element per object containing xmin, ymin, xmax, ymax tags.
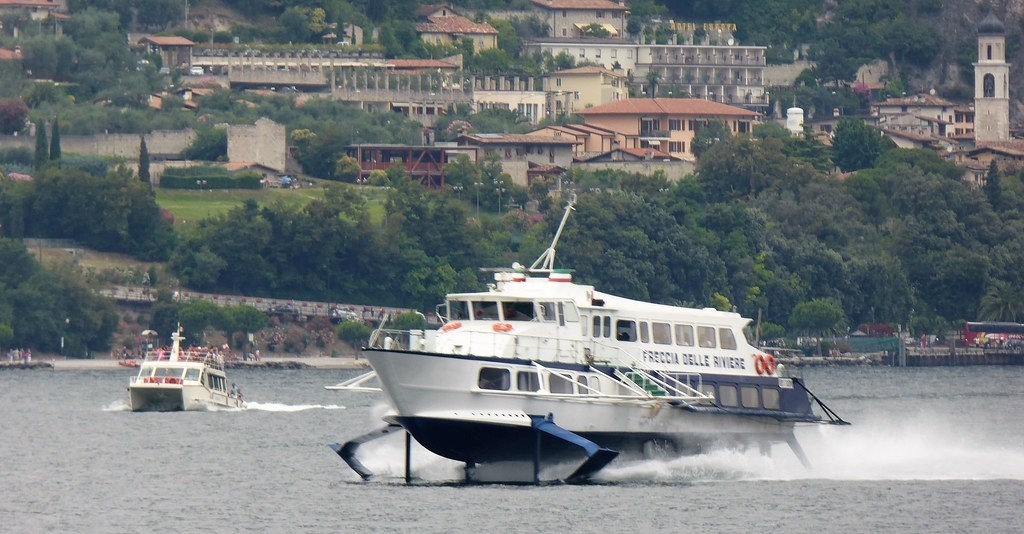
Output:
<box><xmin>125</xmin><ymin>321</ymin><xmax>249</xmax><ymax>412</ymax></box>
<box><xmin>322</xmin><ymin>194</ymin><xmax>851</xmax><ymax>485</ymax></box>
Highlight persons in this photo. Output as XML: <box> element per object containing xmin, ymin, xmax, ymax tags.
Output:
<box><xmin>110</xmin><ymin>344</ymin><xmax>262</xmax><ymax>402</ymax></box>
<box><xmin>383</xmin><ymin>332</ymin><xmax>394</xmax><ymax>350</ymax></box>
<box><xmin>12</xmin><ymin>347</ymin><xmax>32</xmax><ymax>364</ymax></box>
<box><xmin>966</xmin><ymin>336</ymin><xmax>1024</xmax><ymax>349</ymax></box>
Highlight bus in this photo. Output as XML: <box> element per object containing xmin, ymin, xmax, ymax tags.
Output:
<box><xmin>963</xmin><ymin>320</ymin><xmax>1024</xmax><ymax>352</ymax></box>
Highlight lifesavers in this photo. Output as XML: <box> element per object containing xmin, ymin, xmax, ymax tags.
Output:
<box><xmin>494</xmin><ymin>322</ymin><xmax>513</xmax><ymax>332</ymax></box>
<box><xmin>754</xmin><ymin>354</ymin><xmax>776</xmax><ymax>375</ymax></box>
<box><xmin>443</xmin><ymin>321</ymin><xmax>461</xmax><ymax>331</ymax></box>
